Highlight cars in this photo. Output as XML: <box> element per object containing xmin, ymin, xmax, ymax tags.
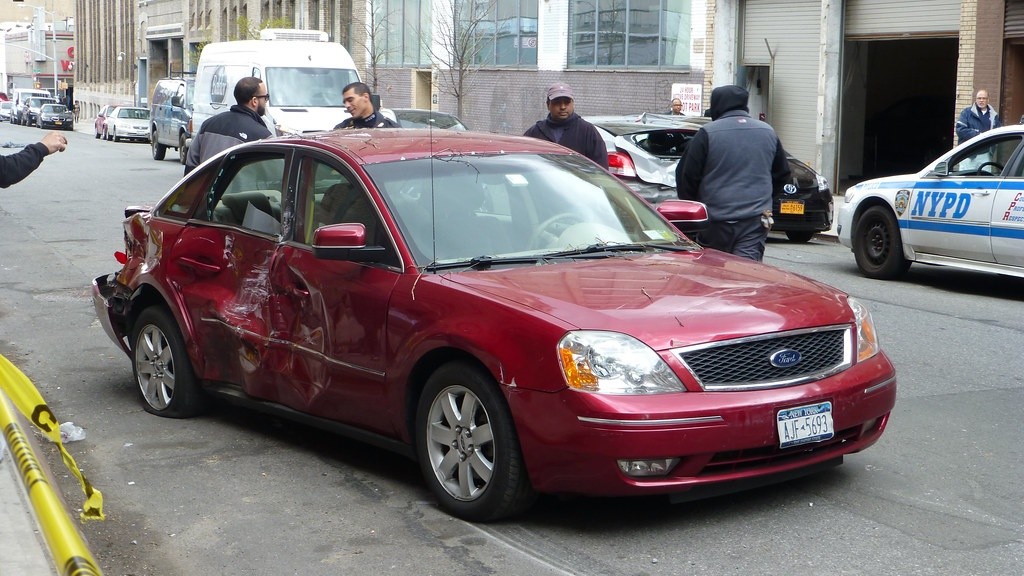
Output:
<box><xmin>0</xmin><ymin>101</ymin><xmax>14</xmax><ymax>122</ymax></box>
<box><xmin>95</xmin><ymin>104</ymin><xmax>151</xmax><ymax>141</ymax></box>
<box><xmin>94</xmin><ymin>128</ymin><xmax>900</xmax><ymax>527</ymax></box>
<box><xmin>37</xmin><ymin>103</ymin><xmax>74</xmax><ymax>131</ymax></box>
<box><xmin>381</xmin><ymin>108</ymin><xmax>473</xmax><ymax>131</ymax></box>
<box><xmin>836</xmin><ymin>126</ymin><xmax>1024</xmax><ymax>285</ymax></box>
<box><xmin>581</xmin><ymin>111</ymin><xmax>833</xmax><ymax>245</ymax></box>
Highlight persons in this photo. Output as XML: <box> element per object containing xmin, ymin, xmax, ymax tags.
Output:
<box><xmin>0</xmin><ymin>131</ymin><xmax>67</xmax><ymax>189</ymax></box>
<box><xmin>316</xmin><ymin>82</ymin><xmax>402</xmax><ymax>180</ymax></box>
<box><xmin>665</xmin><ymin>98</ymin><xmax>685</xmax><ymax>115</ymax></box>
<box><xmin>73</xmin><ymin>100</ymin><xmax>80</xmax><ymax>123</ymax></box>
<box><xmin>675</xmin><ymin>85</ymin><xmax>791</xmax><ymax>260</ymax></box>
<box><xmin>956</xmin><ymin>89</ymin><xmax>1001</xmax><ymax>173</ymax></box>
<box><xmin>523</xmin><ymin>82</ymin><xmax>608</xmax><ymax>170</ymax></box>
<box><xmin>184</xmin><ymin>76</ymin><xmax>287</xmax><ymax>197</ymax></box>
<box><xmin>62</xmin><ymin>98</ymin><xmax>67</xmax><ymax>105</ymax></box>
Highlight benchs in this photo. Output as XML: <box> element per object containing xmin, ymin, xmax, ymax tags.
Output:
<box><xmin>214</xmin><ymin>190</ymin><xmax>325</xmax><ymax>234</ymax></box>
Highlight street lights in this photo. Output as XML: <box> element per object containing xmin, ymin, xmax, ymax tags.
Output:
<box><xmin>17</xmin><ymin>4</ymin><xmax>60</xmax><ymax>99</ymax></box>
<box><xmin>118</xmin><ymin>51</ymin><xmax>127</xmax><ymax>97</ymax></box>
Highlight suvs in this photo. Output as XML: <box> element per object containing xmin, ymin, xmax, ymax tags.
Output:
<box><xmin>21</xmin><ymin>97</ymin><xmax>59</xmax><ymax>127</ymax></box>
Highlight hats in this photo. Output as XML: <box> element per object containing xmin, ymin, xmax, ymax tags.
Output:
<box><xmin>547</xmin><ymin>83</ymin><xmax>575</xmax><ymax>102</ymax></box>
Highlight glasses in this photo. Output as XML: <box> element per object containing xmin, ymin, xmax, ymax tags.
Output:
<box><xmin>673</xmin><ymin>104</ymin><xmax>682</xmax><ymax>106</ymax></box>
<box><xmin>251</xmin><ymin>94</ymin><xmax>269</xmax><ymax>101</ymax></box>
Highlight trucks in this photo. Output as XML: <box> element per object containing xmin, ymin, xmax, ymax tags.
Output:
<box><xmin>11</xmin><ymin>88</ymin><xmax>51</xmax><ymax>124</ymax></box>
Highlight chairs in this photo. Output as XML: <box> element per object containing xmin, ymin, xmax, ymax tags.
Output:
<box><xmin>333</xmin><ymin>185</ymin><xmax>396</xmax><ymax>245</ymax></box>
<box><xmin>646</xmin><ymin>131</ymin><xmax>675</xmax><ymax>156</ymax></box>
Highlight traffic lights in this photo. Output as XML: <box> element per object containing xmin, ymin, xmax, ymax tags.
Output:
<box><xmin>62</xmin><ymin>82</ymin><xmax>69</xmax><ymax>90</ymax></box>
<box><xmin>34</xmin><ymin>82</ymin><xmax>41</xmax><ymax>88</ymax></box>
<box><xmin>57</xmin><ymin>81</ymin><xmax>62</xmax><ymax>91</ymax></box>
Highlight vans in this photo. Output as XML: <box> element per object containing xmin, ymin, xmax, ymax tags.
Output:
<box><xmin>148</xmin><ymin>76</ymin><xmax>196</xmax><ymax>164</ymax></box>
<box><xmin>187</xmin><ymin>27</ymin><xmax>362</xmax><ymax>139</ymax></box>
